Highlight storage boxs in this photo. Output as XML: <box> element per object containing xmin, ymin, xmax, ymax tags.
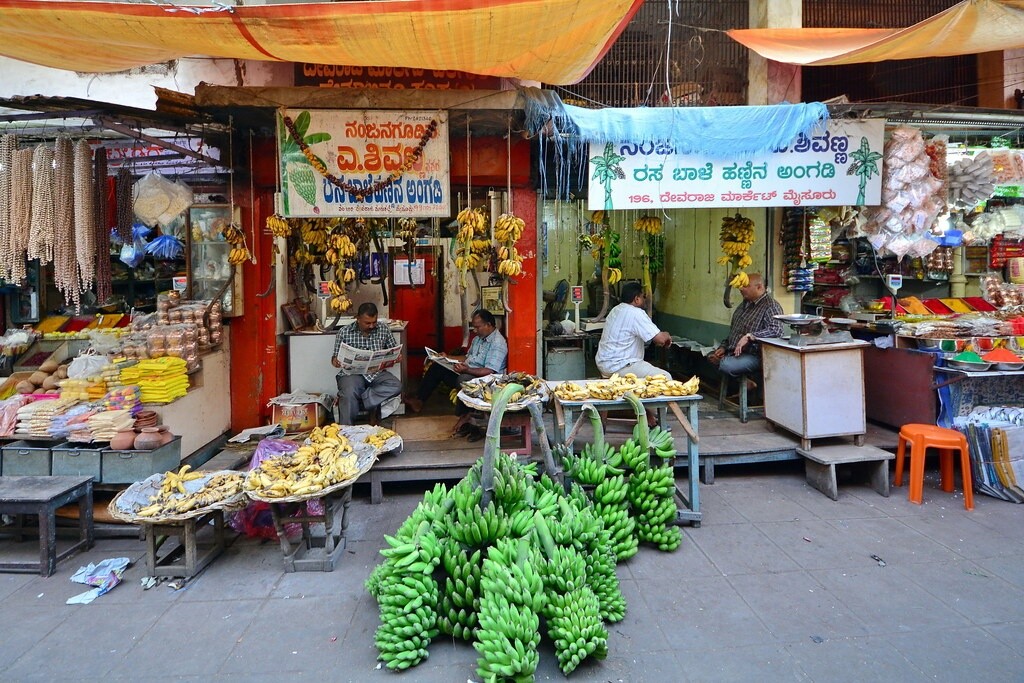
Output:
<box><xmin>272</xmin><ymin>391</ymin><xmax>326</xmax><ymax>434</ymax></box>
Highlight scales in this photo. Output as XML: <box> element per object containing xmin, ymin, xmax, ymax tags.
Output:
<box><xmin>571</xmin><ymin>286</ymin><xmax>607</xmax><ymax>333</ymax></box>
<box><xmin>856</xmin><ymin>274</ymin><xmax>903</xmax><ymax>321</ymax></box>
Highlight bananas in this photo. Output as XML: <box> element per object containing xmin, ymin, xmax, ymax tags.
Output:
<box><xmin>222</xmin><ymin>222</ymin><xmax>248</xmax><ymax>265</ymax></box>
<box><xmin>265</xmin><ymin>207</ymin><xmax>527</xmax><ymax>312</ymax></box>
<box><xmin>449</xmin><ymin>370</ymin><xmax>541</xmax><ymax>404</ymax></box>
<box><xmin>367</xmin><ymin>423</ymin><xmax>682</xmax><ymax>683</ymax></box>
<box><xmin>577</xmin><ymin>210</ymin><xmax>755</xmax><ymax>289</ymax></box>
<box><xmin>552</xmin><ymin>373</ymin><xmax>700</xmax><ymax>400</ymax></box>
<box><xmin>136</xmin><ymin>423</ymin><xmax>396</xmax><ymax>515</ymax></box>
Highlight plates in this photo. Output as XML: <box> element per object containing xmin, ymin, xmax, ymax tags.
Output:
<box><xmin>134</xmin><ymin>411</ymin><xmax>156</xmax><ymax>430</ymax></box>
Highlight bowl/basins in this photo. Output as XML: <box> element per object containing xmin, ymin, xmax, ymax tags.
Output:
<box><xmin>942</xmin><ymin>357</ymin><xmax>997</xmax><ymax>371</ymax></box>
<box><xmin>982</xmin><ymin>359</ymin><xmax>1024</xmax><ymax>370</ymax></box>
<box><xmin>917</xmin><ymin>336</ymin><xmax>1024</xmax><ymax>353</ymax></box>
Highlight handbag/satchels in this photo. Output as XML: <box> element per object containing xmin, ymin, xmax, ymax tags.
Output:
<box><xmin>951</xmin><ymin>405</ymin><xmax>1024</xmax><ymax>504</ymax></box>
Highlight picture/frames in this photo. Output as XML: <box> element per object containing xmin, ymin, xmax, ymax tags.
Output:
<box><xmin>281</xmin><ymin>302</ymin><xmax>306</xmax><ymax>331</ymax></box>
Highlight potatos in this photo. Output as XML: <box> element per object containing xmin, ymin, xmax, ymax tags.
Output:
<box><xmin>16</xmin><ymin>361</ymin><xmax>69</xmax><ymax>396</ymax></box>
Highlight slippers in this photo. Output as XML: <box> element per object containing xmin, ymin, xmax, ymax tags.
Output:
<box><xmin>455</xmin><ymin>423</ymin><xmax>481</xmax><ymax>437</ymax></box>
<box><xmin>467</xmin><ymin>426</ymin><xmax>486</xmax><ymax>442</ymax></box>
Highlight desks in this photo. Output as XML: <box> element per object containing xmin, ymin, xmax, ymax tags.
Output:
<box><xmin>756</xmin><ymin>336</ymin><xmax>871</xmax><ymax>449</ymax></box>
<box><xmin>288</xmin><ymin>320</ymin><xmax>408</xmax><ymax>414</ymax></box>
<box><xmin>0</xmin><ymin>476</ymin><xmax>95</xmax><ymax>578</ymax></box>
<box><xmin>543</xmin><ymin>334</ymin><xmax>594</xmax><ymax>381</ymax></box>
<box><xmin>545</xmin><ymin>380</ymin><xmax>702</xmax><ymax>528</ymax></box>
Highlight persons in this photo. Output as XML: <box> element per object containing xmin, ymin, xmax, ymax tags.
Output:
<box><xmin>331</xmin><ymin>302</ymin><xmax>402</xmax><ymax>426</ymax></box>
<box><xmin>595</xmin><ymin>282</ymin><xmax>660</xmax><ymax>420</ymax></box>
<box><xmin>401</xmin><ymin>309</ymin><xmax>508</xmax><ymax>431</ymax></box>
<box><xmin>708</xmin><ymin>273</ymin><xmax>784</xmax><ymax>389</ymax></box>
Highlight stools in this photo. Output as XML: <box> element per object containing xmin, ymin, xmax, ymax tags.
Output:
<box><xmin>146</xmin><ymin>508</ymin><xmax>225</xmax><ymax>578</ymax></box>
<box><xmin>895</xmin><ymin>423</ymin><xmax>975</xmax><ymax>511</ymax></box>
<box><xmin>483</xmin><ymin>411</ymin><xmax>531</xmax><ymax>455</ymax></box>
<box><xmin>717</xmin><ymin>372</ymin><xmax>765</xmax><ymax>422</ymax></box>
<box><xmin>269</xmin><ymin>483</ymin><xmax>354</xmax><ymax>572</ymax></box>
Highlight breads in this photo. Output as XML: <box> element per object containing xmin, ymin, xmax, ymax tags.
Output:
<box><xmin>108</xmin><ymin>292</ymin><xmax>222</xmax><ymax>371</ymax></box>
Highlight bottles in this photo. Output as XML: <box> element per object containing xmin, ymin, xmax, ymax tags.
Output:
<box><xmin>110</xmin><ymin>425</ymin><xmax>174</xmax><ymax>450</ymax></box>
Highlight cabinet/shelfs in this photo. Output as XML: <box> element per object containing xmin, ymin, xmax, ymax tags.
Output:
<box><xmin>184</xmin><ymin>202</ymin><xmax>244</xmax><ymax>318</ymax></box>
<box><xmin>108</xmin><ymin>252</ymin><xmax>186</xmax><ymax>310</ymax></box>
<box><xmin>804</xmin><ymin>241</ymin><xmax>992</xmax><ymax>310</ymax></box>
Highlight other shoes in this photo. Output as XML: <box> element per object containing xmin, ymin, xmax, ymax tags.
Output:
<box><xmin>649</xmin><ymin>420</ymin><xmax>671</xmax><ymax>432</ymax></box>
<box><xmin>500</xmin><ymin>426</ymin><xmax>520</xmax><ymax>437</ymax></box>
